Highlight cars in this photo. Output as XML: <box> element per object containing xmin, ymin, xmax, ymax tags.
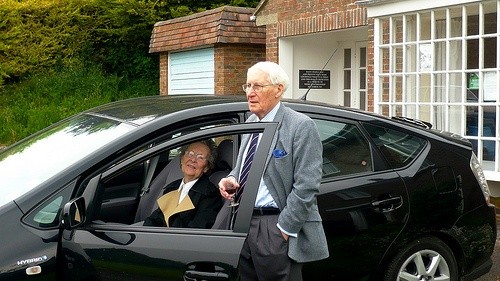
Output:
<box><xmin>1</xmin><ymin>94</ymin><xmax>497</xmax><ymax>281</ymax></box>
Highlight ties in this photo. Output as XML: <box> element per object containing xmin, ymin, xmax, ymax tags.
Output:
<box><xmin>235</xmin><ymin>133</ymin><xmax>259</xmax><ymax>215</ymax></box>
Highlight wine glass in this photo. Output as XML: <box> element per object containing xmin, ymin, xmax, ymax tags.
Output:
<box><xmin>222</xmin><ymin>176</ymin><xmax>239</xmax><ymax>207</ymax></box>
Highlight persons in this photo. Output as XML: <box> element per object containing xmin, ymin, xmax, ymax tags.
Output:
<box><xmin>218</xmin><ymin>61</ymin><xmax>330</xmax><ymax>281</ymax></box>
<box><xmin>142</xmin><ymin>137</ymin><xmax>222</xmax><ymax>229</ymax></box>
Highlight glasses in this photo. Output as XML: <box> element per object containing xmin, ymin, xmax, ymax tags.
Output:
<box><xmin>185</xmin><ymin>151</ymin><xmax>206</xmax><ymax>162</ymax></box>
<box><xmin>242</xmin><ymin>83</ymin><xmax>273</xmax><ymax>93</ymax></box>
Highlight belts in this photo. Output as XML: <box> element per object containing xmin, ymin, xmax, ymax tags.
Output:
<box><xmin>253</xmin><ymin>208</ymin><xmax>280</xmax><ymax>215</ymax></box>
